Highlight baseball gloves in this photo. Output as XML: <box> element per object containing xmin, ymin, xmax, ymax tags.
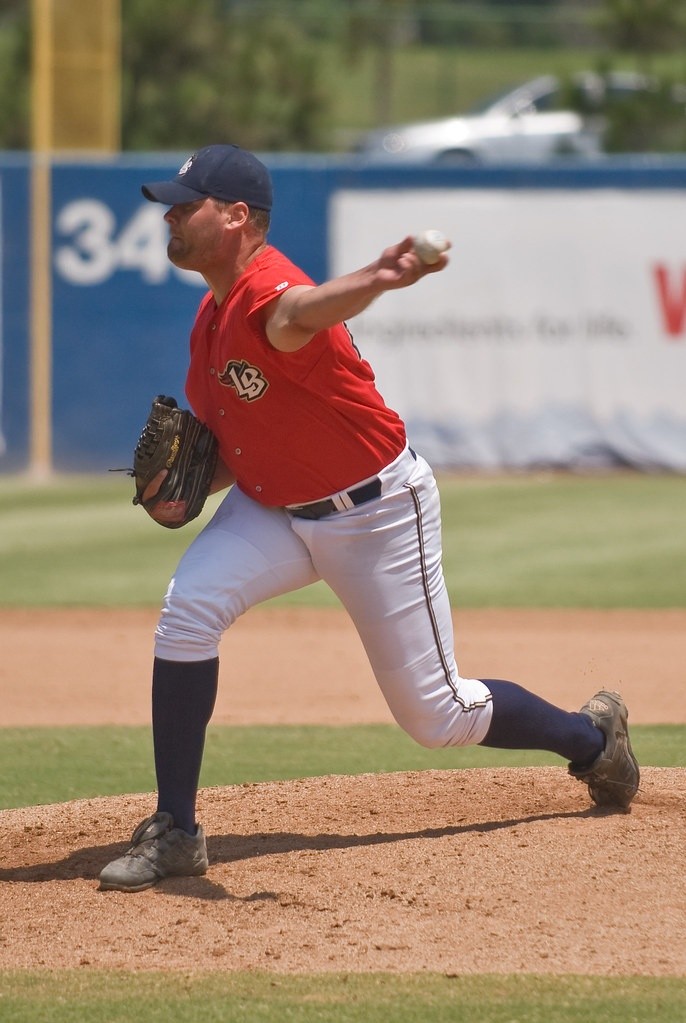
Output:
<box><xmin>107</xmin><ymin>393</ymin><xmax>222</xmax><ymax>531</ymax></box>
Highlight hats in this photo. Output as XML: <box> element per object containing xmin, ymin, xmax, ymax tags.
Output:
<box><xmin>141</xmin><ymin>144</ymin><xmax>273</xmax><ymax>211</ymax></box>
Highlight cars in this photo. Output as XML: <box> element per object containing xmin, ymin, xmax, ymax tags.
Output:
<box><xmin>354</xmin><ymin>70</ymin><xmax>686</xmax><ymax>174</ymax></box>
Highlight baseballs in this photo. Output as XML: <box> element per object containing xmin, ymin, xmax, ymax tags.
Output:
<box><xmin>414</xmin><ymin>227</ymin><xmax>448</xmax><ymax>267</ymax></box>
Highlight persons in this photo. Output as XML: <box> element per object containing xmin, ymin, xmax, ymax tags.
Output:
<box><xmin>97</xmin><ymin>143</ymin><xmax>641</xmax><ymax>894</ymax></box>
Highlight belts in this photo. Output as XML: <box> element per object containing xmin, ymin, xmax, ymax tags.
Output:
<box><xmin>288</xmin><ymin>446</ymin><xmax>417</xmax><ymax>520</ymax></box>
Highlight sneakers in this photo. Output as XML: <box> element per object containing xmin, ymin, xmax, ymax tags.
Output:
<box><xmin>99</xmin><ymin>811</ymin><xmax>209</xmax><ymax>893</ymax></box>
<box><xmin>568</xmin><ymin>690</ymin><xmax>640</xmax><ymax>808</ymax></box>
